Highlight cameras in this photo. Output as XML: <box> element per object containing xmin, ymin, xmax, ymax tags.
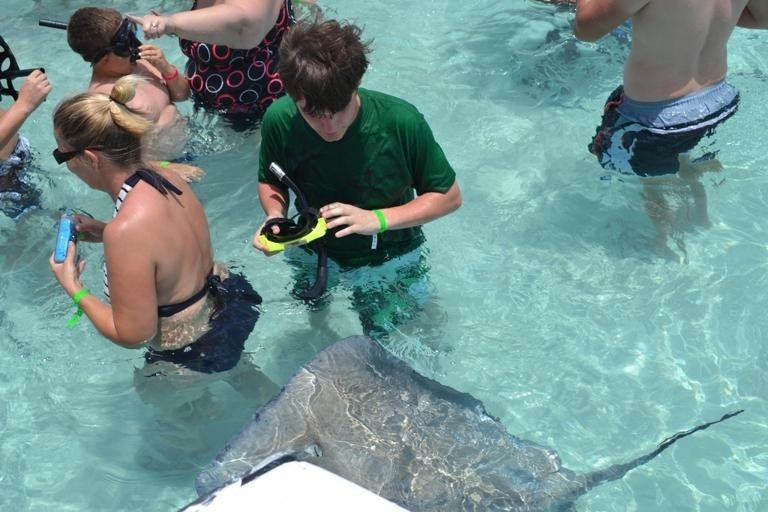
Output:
<box><xmin>54</xmin><ymin>218</ymin><xmax>79</xmax><ymax>263</ymax></box>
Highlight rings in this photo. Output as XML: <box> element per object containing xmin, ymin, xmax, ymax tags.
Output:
<box><xmin>152</xmin><ymin>22</ymin><xmax>161</xmax><ymax>30</ymax></box>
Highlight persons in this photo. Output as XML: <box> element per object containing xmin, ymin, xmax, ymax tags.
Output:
<box><xmin>68</xmin><ymin>6</ymin><xmax>217</xmax><ymax>185</ymax></box>
<box><xmin>129</xmin><ymin>1</ymin><xmax>313</xmax><ymax>140</ymax></box>
<box><xmin>249</xmin><ymin>8</ymin><xmax>465</xmax><ymax>371</ymax></box>
<box><xmin>519</xmin><ymin>1</ymin><xmax>633</xmax><ymax>103</ymax></box>
<box><xmin>48</xmin><ymin>73</ymin><xmax>277</xmax><ymax>471</ymax></box>
<box><xmin>1</xmin><ymin>68</ymin><xmax>61</xmax><ymax>267</ymax></box>
<box><xmin>567</xmin><ymin>1</ymin><xmax>768</xmax><ymax>260</ymax></box>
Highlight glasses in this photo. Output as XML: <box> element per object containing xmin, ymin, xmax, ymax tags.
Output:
<box><xmin>84</xmin><ymin>17</ymin><xmax>138</xmax><ymax>63</ymax></box>
<box><xmin>52</xmin><ymin>147</ymin><xmax>82</xmax><ymax>165</ymax></box>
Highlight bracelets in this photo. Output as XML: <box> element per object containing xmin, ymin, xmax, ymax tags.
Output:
<box><xmin>375</xmin><ymin>209</ymin><xmax>390</xmax><ymax>233</ymax></box>
<box><xmin>162</xmin><ymin>64</ymin><xmax>179</xmax><ymax>82</ymax></box>
<box><xmin>68</xmin><ymin>288</ymin><xmax>92</xmax><ymax>324</ymax></box>
